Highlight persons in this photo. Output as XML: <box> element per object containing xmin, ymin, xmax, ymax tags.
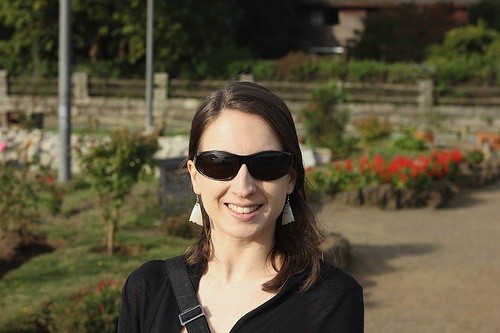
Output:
<box><xmin>118</xmin><ymin>81</ymin><xmax>364</xmax><ymax>333</ymax></box>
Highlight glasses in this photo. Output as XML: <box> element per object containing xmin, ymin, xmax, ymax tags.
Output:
<box><xmin>194</xmin><ymin>150</ymin><xmax>294</xmax><ymax>181</ymax></box>
